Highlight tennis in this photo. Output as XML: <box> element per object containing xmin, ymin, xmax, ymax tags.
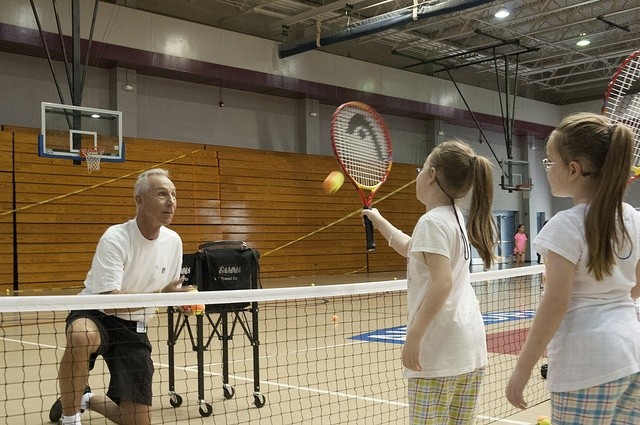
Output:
<box><xmin>322</xmin><ymin>171</ymin><xmax>345</xmax><ymax>193</ymax></box>
<box><xmin>537</xmin><ymin>415</ymin><xmax>551</xmax><ymax>425</ymax></box>
<box><xmin>332</xmin><ymin>315</ymin><xmax>338</xmax><ymax>321</ymax></box>
<box><xmin>394</xmin><ymin>277</ymin><xmax>397</xmax><ymax>280</ymax></box>
<box><xmin>311</xmin><ymin>282</ymin><xmax>315</xmax><ymax>287</ymax></box>
<box><xmin>191</xmin><ymin>303</ymin><xmax>205</xmax><ymax>315</ymax></box>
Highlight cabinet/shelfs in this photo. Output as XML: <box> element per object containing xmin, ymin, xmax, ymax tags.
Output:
<box><xmin>167</xmin><ymin>252</ymin><xmax>265</xmax><ymax>416</ymax></box>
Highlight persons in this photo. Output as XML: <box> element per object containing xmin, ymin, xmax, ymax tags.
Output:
<box><xmin>514</xmin><ymin>222</ymin><xmax>528</xmax><ymax>266</ymax></box>
<box><xmin>360</xmin><ymin>139</ymin><xmax>496</xmax><ymax>425</ymax></box>
<box><xmin>504</xmin><ymin>110</ymin><xmax>640</xmax><ymax>425</ymax></box>
<box><xmin>49</xmin><ymin>169</ymin><xmax>195</xmax><ymax>425</ymax></box>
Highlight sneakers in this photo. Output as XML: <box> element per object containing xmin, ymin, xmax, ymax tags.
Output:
<box><xmin>49</xmin><ymin>384</ymin><xmax>91</xmax><ymax>423</ymax></box>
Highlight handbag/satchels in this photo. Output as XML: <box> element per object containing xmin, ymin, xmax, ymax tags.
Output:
<box><xmin>193</xmin><ymin>240</ymin><xmax>263</xmax><ymax>311</ymax></box>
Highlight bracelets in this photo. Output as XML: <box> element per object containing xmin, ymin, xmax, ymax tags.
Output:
<box><xmin>158</xmin><ymin>289</ymin><xmax>162</xmax><ymax>293</ymax></box>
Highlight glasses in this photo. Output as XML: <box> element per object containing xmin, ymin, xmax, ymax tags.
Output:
<box><xmin>541</xmin><ymin>156</ymin><xmax>583</xmax><ymax>175</ymax></box>
<box><xmin>416</xmin><ymin>168</ymin><xmax>432</xmax><ymax>174</ymax></box>
